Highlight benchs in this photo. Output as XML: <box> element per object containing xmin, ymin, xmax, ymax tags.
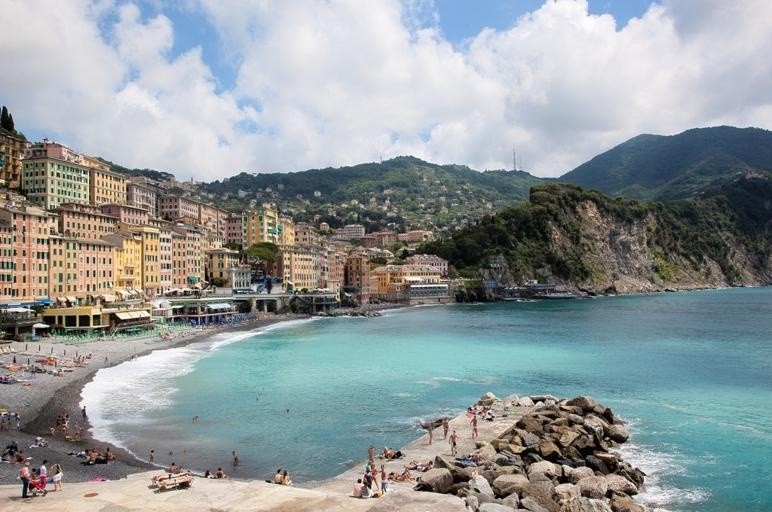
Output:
<box><xmin>152</xmin><ymin>472</ymin><xmax>192</xmax><ymax>494</ymax></box>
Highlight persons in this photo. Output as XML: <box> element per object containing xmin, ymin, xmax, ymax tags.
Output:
<box><xmin>145</xmin><ymin>414</ymin><xmax>294</xmax><ymax>491</ymax></box>
<box><xmin>350</xmin><ymin>400</ymin><xmax>513</xmax><ymax>500</ymax></box>
<box><xmin>1</xmin><ymin>344</ymin><xmax>118</xmax><ymax>499</ymax></box>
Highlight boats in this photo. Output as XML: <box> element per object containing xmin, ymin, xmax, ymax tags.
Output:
<box><xmin>498</xmin><ymin>282</ymin><xmax>579</xmax><ymax>302</ymax></box>
